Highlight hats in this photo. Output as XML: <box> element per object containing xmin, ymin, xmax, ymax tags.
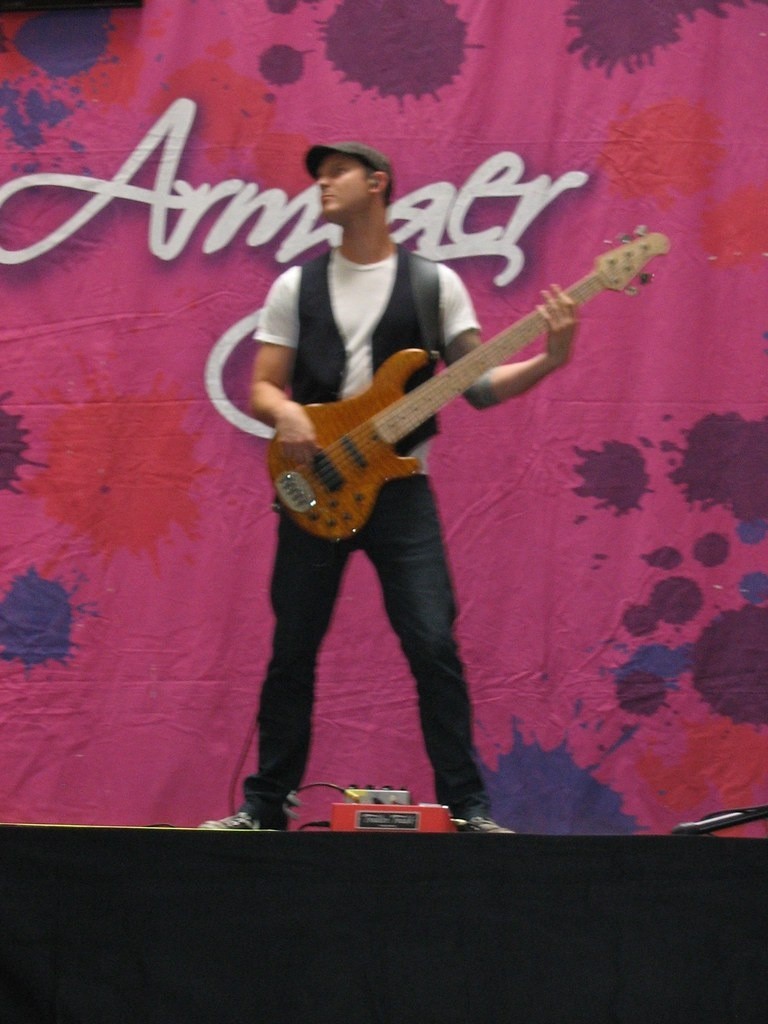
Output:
<box><xmin>305</xmin><ymin>141</ymin><xmax>391</xmax><ymax>194</ymax></box>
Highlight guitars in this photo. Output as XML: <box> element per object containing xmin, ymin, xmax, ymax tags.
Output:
<box><xmin>264</xmin><ymin>223</ymin><xmax>669</xmax><ymax>541</ymax></box>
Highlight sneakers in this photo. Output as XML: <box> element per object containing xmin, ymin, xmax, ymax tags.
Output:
<box><xmin>197</xmin><ymin>811</ymin><xmax>260</xmax><ymax>829</ymax></box>
<box><xmin>457</xmin><ymin>817</ymin><xmax>514</xmax><ymax>833</ymax></box>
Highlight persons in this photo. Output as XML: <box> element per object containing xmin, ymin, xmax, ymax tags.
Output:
<box><xmin>195</xmin><ymin>140</ymin><xmax>583</xmax><ymax>835</ymax></box>
